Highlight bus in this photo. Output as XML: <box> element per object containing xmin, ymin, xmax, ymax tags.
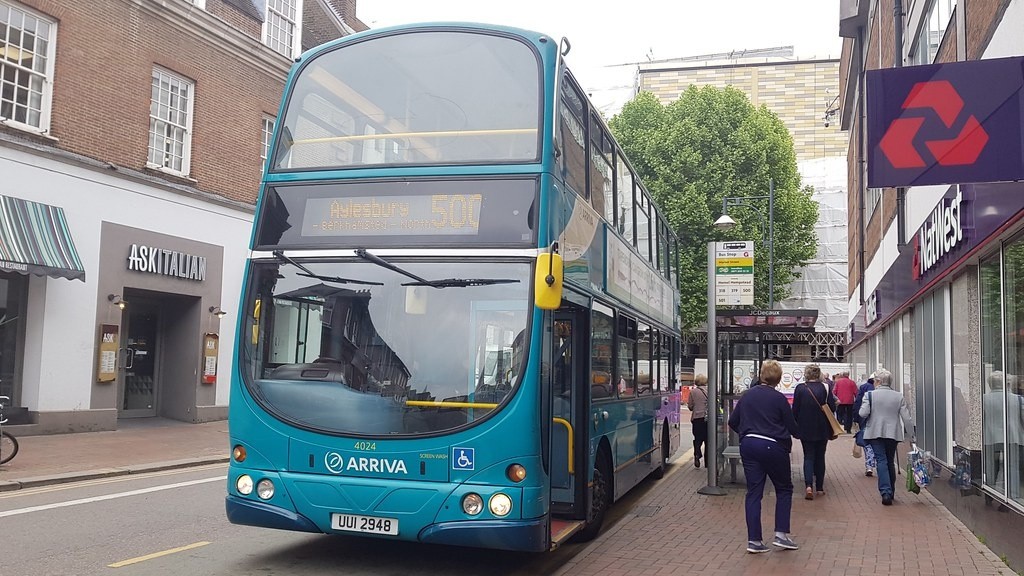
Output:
<box><xmin>224</xmin><ymin>20</ymin><xmax>681</xmax><ymax>556</ymax></box>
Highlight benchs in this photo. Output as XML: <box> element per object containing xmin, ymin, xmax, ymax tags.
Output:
<box><xmin>721</xmin><ymin>446</ymin><xmax>742</xmax><ymax>485</ymax></box>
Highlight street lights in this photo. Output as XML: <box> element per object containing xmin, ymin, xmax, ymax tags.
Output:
<box><xmin>712</xmin><ymin>178</ymin><xmax>772</xmax><ymax>360</ymax></box>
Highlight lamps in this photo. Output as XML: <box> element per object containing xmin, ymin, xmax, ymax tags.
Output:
<box><xmin>108</xmin><ymin>294</ymin><xmax>128</xmax><ymax>310</ymax></box>
<box><xmin>210</xmin><ymin>306</ymin><xmax>227</xmax><ymax>318</ymax></box>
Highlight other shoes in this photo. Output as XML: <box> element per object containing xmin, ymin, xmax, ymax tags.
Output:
<box><xmin>816</xmin><ymin>490</ymin><xmax>825</xmax><ymax>497</ymax></box>
<box><xmin>694</xmin><ymin>455</ymin><xmax>700</xmax><ymax>468</ymax></box>
<box><xmin>746</xmin><ymin>539</ymin><xmax>771</xmax><ymax>553</ymax></box>
<box><xmin>882</xmin><ymin>493</ymin><xmax>892</xmax><ymax>506</ymax></box>
<box><xmin>864</xmin><ymin>467</ymin><xmax>873</xmax><ymax>476</ymax></box>
<box><xmin>772</xmin><ymin>534</ymin><xmax>798</xmax><ymax>550</ymax></box>
<box><xmin>804</xmin><ymin>485</ymin><xmax>814</xmax><ymax>500</ymax></box>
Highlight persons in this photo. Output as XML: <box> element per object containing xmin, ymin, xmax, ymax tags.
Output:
<box><xmin>687</xmin><ymin>374</ymin><xmax>708</xmax><ymax>468</ymax></box>
<box><xmin>859</xmin><ymin>369</ymin><xmax>917</xmax><ymax>505</ymax></box>
<box><xmin>793</xmin><ymin>364</ymin><xmax>835</xmax><ymax>499</ymax></box>
<box><xmin>727</xmin><ymin>361</ymin><xmax>799</xmax><ymax>551</ymax></box>
<box><xmin>832</xmin><ymin>371</ymin><xmax>858</xmax><ymax>433</ymax></box>
<box><xmin>750</xmin><ymin>372</ymin><xmax>759</xmax><ymax>387</ymax></box>
<box><xmin>591</xmin><ymin>371</ymin><xmax>654</xmax><ymax>461</ymax></box>
<box><xmin>823</xmin><ymin>373</ymin><xmax>832</xmax><ymax>387</ymax></box>
<box><xmin>985</xmin><ymin>371</ymin><xmax>1024</xmax><ymax>511</ymax></box>
<box><xmin>854</xmin><ymin>379</ymin><xmax>875</xmax><ymax>476</ymax></box>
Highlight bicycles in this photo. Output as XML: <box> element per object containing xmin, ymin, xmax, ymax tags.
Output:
<box><xmin>0</xmin><ymin>396</ymin><xmax>18</xmax><ymax>466</ymax></box>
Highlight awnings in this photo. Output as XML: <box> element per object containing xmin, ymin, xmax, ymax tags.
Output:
<box><xmin>0</xmin><ymin>194</ymin><xmax>85</xmax><ymax>282</ymax></box>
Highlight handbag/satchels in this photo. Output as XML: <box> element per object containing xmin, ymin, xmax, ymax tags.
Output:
<box><xmin>852</xmin><ymin>441</ymin><xmax>862</xmax><ymax>458</ymax></box>
<box><xmin>853</xmin><ymin>430</ymin><xmax>869</xmax><ymax>447</ymax></box>
<box><xmin>906</xmin><ymin>451</ymin><xmax>920</xmax><ymax>496</ymax></box>
<box><xmin>911</xmin><ymin>443</ymin><xmax>933</xmax><ymax>488</ymax></box>
<box><xmin>822</xmin><ymin>404</ymin><xmax>845</xmax><ymax>436</ymax></box>
